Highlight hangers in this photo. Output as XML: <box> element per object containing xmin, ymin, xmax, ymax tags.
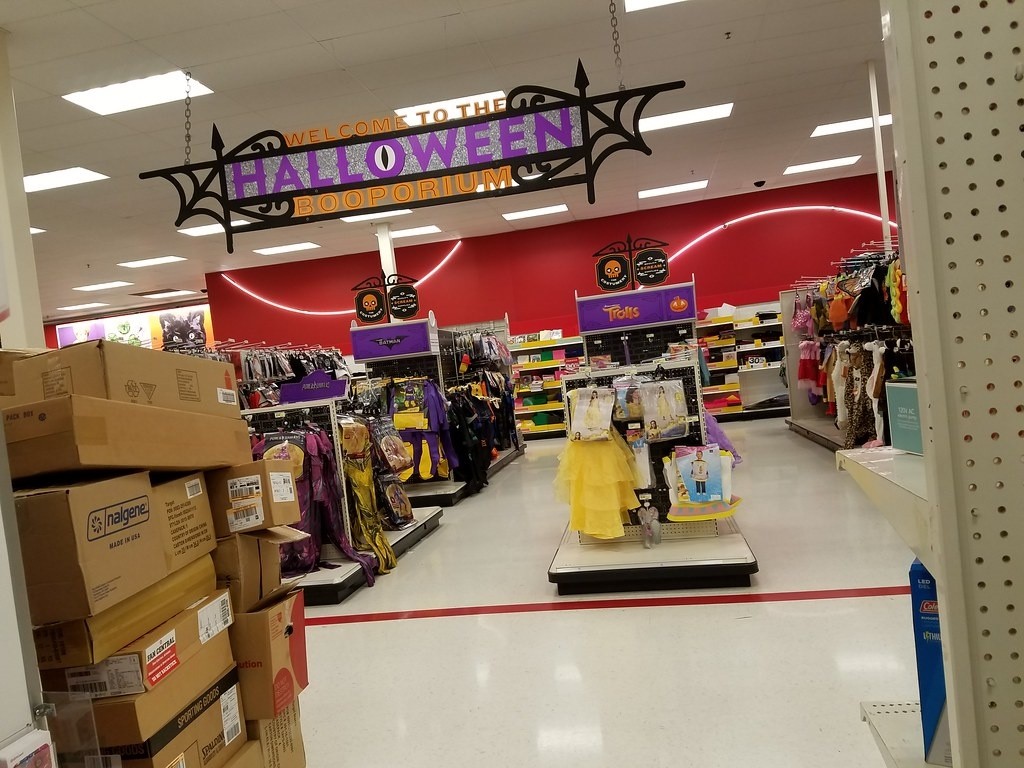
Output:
<box><xmin>794</xmin><ymin>251</ymin><xmax>900</xmax><ymax>298</ymax></box>
<box><xmin>804</xmin><ymin>326</ymin><xmax>909</xmax><ymax>347</ymax></box>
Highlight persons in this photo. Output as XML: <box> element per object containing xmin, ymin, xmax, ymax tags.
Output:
<box><xmin>583</xmin><ymin>390</ymin><xmax>603</xmax><ymax>431</ymax></box>
<box><xmin>656</xmin><ymin>386</ymin><xmax>674</xmax><ymax>420</ymax></box>
<box><xmin>625</xmin><ymin>388</ymin><xmax>645</xmax><ymax>417</ymax></box>
<box><xmin>647</xmin><ymin>420</ymin><xmax>661</xmax><ymax>441</ymax></box>
<box><xmin>574</xmin><ymin>432</ymin><xmax>580</xmax><ymax>440</ymax></box>
<box><xmin>485</xmin><ymin>338</ymin><xmax>497</xmax><ymax>355</ymax></box>
<box><xmin>690</xmin><ymin>451</ymin><xmax>709</xmax><ymax>495</ymax></box>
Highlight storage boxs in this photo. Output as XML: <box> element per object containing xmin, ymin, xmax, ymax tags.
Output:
<box><xmin>885</xmin><ymin>376</ymin><xmax>924</xmax><ymax>457</ymax></box>
<box><xmin>909</xmin><ymin>556</ymin><xmax>954</xmax><ymax>768</ymax></box>
<box><xmin>0</xmin><ymin>340</ymin><xmax>309</xmax><ymax>768</ymax></box>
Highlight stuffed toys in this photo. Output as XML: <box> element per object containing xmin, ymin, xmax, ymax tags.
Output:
<box><xmin>637</xmin><ymin>507</ymin><xmax>661</xmax><ymax>548</ymax></box>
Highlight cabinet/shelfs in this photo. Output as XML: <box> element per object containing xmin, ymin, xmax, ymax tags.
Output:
<box><xmin>508</xmin><ymin>341</ymin><xmax>585</xmax><ymax>434</ymax></box>
<box><xmin>696</xmin><ymin>322</ymin><xmax>790</xmax><ymax>416</ymax></box>
<box><xmin>835</xmin><ymin>1</ymin><xmax>1024</xmax><ymax>768</ymax></box>
